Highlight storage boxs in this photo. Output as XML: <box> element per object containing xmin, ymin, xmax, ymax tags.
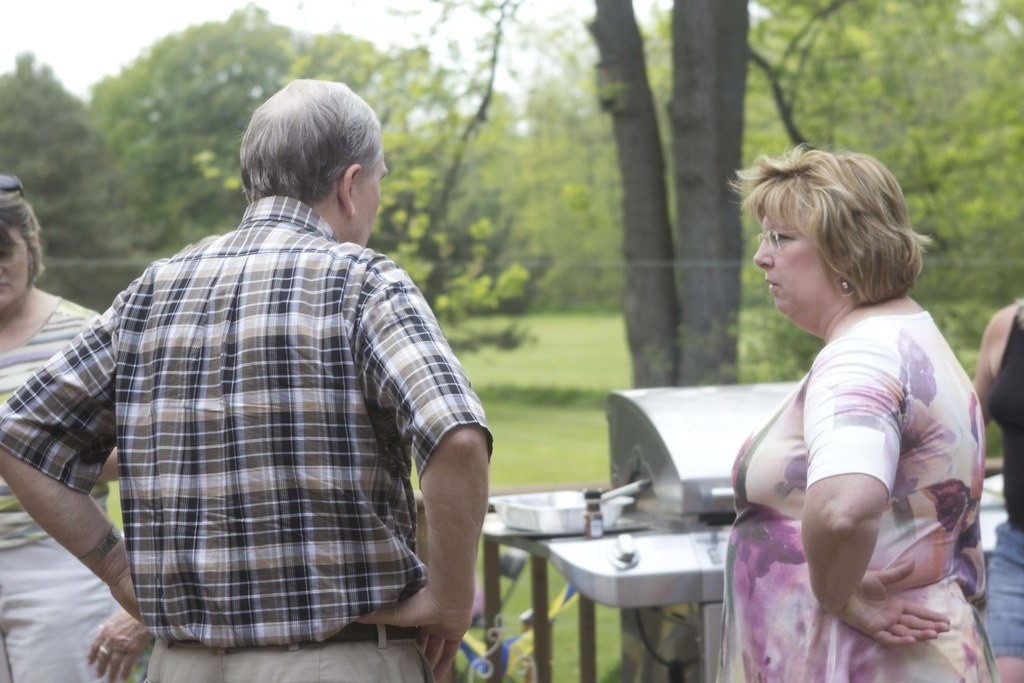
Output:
<box><xmin>498</xmin><ymin>488</ymin><xmax>634</xmax><ymax>529</ymax></box>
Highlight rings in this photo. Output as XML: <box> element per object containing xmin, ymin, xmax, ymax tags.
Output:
<box><xmin>99</xmin><ymin>646</ymin><xmax>110</xmax><ymax>656</ymax></box>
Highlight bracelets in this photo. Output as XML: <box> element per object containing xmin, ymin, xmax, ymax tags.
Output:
<box><xmin>78</xmin><ymin>524</ymin><xmax>122</xmax><ymax>568</ymax></box>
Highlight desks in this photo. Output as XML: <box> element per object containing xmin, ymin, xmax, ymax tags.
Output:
<box><xmin>549</xmin><ymin>507</ymin><xmax>1010</xmax><ymax>683</ymax></box>
<box><xmin>480</xmin><ymin>493</ymin><xmax>656</xmax><ymax>683</ymax></box>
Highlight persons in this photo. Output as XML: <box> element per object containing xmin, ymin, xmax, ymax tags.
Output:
<box><xmin>714</xmin><ymin>144</ymin><xmax>1000</xmax><ymax>683</ymax></box>
<box><xmin>972</xmin><ymin>298</ymin><xmax>1024</xmax><ymax>682</ymax></box>
<box><xmin>0</xmin><ymin>78</ymin><xmax>493</xmax><ymax>682</ymax></box>
<box><xmin>0</xmin><ymin>173</ymin><xmax>132</xmax><ymax>682</ymax></box>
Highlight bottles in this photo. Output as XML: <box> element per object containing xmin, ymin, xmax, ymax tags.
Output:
<box><xmin>585</xmin><ymin>491</ymin><xmax>603</xmax><ymax>540</ymax></box>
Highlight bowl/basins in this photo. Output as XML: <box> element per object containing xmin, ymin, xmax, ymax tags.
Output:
<box><xmin>488</xmin><ymin>491</ymin><xmax>634</xmax><ymax>532</ymax></box>
